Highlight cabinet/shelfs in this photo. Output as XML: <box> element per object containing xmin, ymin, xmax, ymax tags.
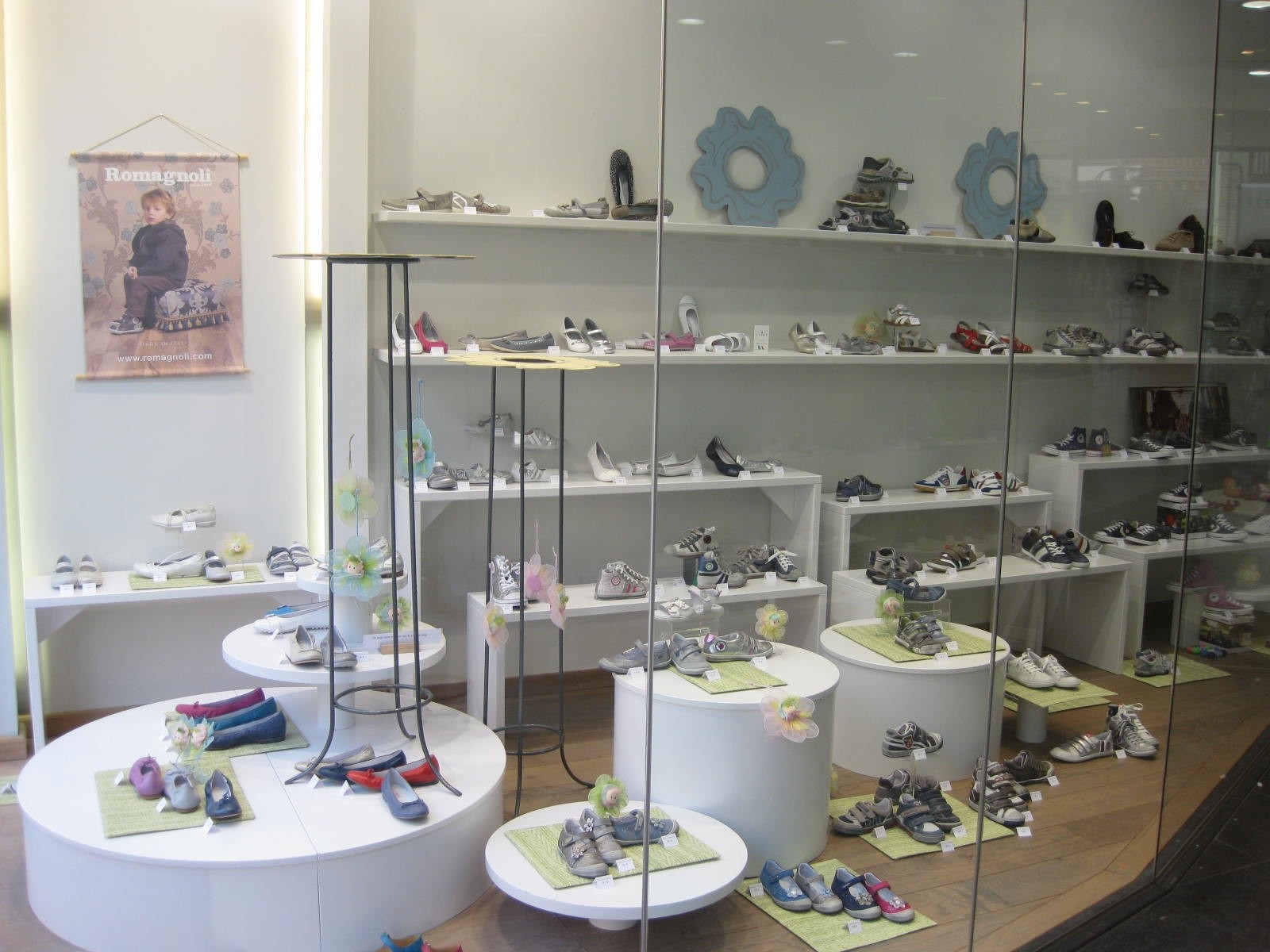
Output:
<box><xmin>19</xmin><ymin>566</ymin><xmax>508</xmax><ymax>952</ymax></box>
<box><xmin>373</xmin><ymin>211</ymin><xmax>1270</xmax><ymax>364</ymax></box>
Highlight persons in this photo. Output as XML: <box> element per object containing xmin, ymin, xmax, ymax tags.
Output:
<box><xmin>110</xmin><ymin>189</ymin><xmax>189</xmax><ymax>335</ymax></box>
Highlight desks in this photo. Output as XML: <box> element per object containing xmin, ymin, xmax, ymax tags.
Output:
<box><xmin>396</xmin><ymin>449</ymin><xmax>1270</xmax><ymax>930</ymax></box>
<box><xmin>24</xmin><ymin>561</ymin><xmax>300</xmax><ymax>753</ymax></box>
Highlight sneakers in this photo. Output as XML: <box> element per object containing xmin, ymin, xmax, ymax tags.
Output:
<box><xmin>970</xmin><ymin>750</ymin><xmax>1055</xmax><ymax>826</ymax></box>
<box><xmin>109</xmin><ymin>313</ymin><xmax>144</xmax><ymax>334</ymax></box>
<box><xmin>408</xmin><ymin>422</ymin><xmax>1269</xmax><ymax>614</ymax></box>
<box><xmin>1051</xmin><ymin>702</ymin><xmax>1159</xmax><ymax>762</ymax></box>
<box><xmin>832</xmin><ymin>721</ymin><xmax>962</xmax><ymax>844</ymax></box>
<box><xmin>1007</xmin><ymin>649</ymin><xmax>1082</xmax><ymax>688</ymax></box>
<box><xmin>896</xmin><ymin>609</ymin><xmax>950</xmax><ymax>655</ymax></box>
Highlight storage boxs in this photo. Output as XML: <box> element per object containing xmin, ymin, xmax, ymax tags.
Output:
<box><xmin>1157</xmin><ymin>501</ymin><xmax>1209</xmax><ymax>540</ymax></box>
<box><xmin>1199</xmin><ymin>611</ymin><xmax>1255</xmax><ymax>653</ymax></box>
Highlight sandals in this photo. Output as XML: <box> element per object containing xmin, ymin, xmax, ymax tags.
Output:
<box><xmin>391</xmin><ymin>301</ymin><xmax>1270</xmax><ymax>360</ymax></box>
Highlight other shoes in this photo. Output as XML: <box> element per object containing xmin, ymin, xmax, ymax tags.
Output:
<box><xmin>1134</xmin><ymin>649</ymin><xmax>1173</xmax><ymax>676</ymax></box>
<box><xmin>381</xmin><ymin>932</ymin><xmax>464</xmax><ymax>952</ymax></box>
<box><xmin>265</xmin><ymin>541</ymin><xmax>313</xmax><ymax>574</ymax></box>
<box><xmin>254</xmin><ymin>599</ymin><xmax>357</xmax><ymax>669</ymax></box>
<box><xmin>129</xmin><ymin>756</ymin><xmax>243</xmax><ymax>819</ymax></box>
<box><xmin>598</xmin><ymin>630</ymin><xmax>774</xmax><ymax>675</ymax></box>
<box><xmin>376</xmin><ymin>149</ymin><xmax>1270</xmax><ymax>256</ymax></box>
<box><xmin>760</xmin><ymin>860</ymin><xmax>916</xmax><ymax>922</ymax></box>
<box><xmin>150</xmin><ymin>506</ymin><xmax>217</xmax><ymax>529</ymax></box>
<box><xmin>175</xmin><ymin>686</ymin><xmax>286</xmax><ymax>751</ymax></box>
<box><xmin>48</xmin><ymin>552</ymin><xmax>104</xmax><ymax>586</ymax></box>
<box><xmin>132</xmin><ymin>548</ymin><xmax>231</xmax><ymax>581</ymax></box>
<box><xmin>557</xmin><ymin>807</ymin><xmax>679</xmax><ymax>876</ymax></box>
<box><xmin>293</xmin><ymin>745</ymin><xmax>440</xmax><ymax>819</ymax></box>
